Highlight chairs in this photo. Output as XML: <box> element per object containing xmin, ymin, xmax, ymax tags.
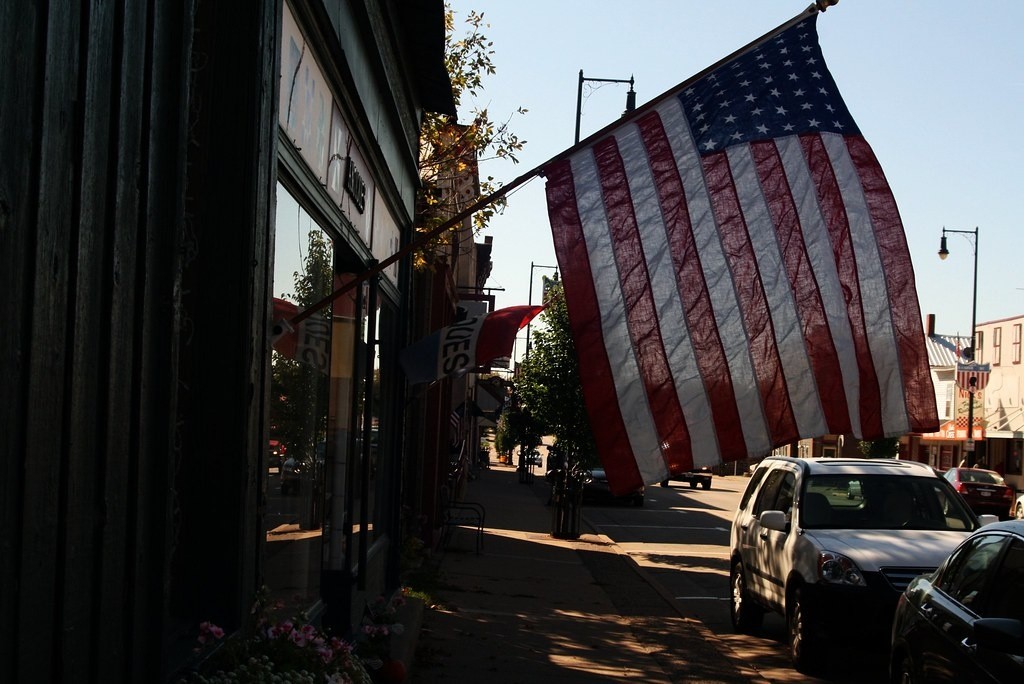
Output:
<box><xmin>802</xmin><ymin>492</ymin><xmax>833</xmax><ymax>529</ymax></box>
<box><xmin>443</xmin><ymin>482</ymin><xmax>485</xmax><ymax>545</ymax></box>
<box><xmin>858</xmin><ymin>494</ymin><xmax>899</xmax><ymax>526</ymax></box>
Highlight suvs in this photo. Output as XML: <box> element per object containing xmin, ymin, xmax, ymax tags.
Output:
<box><xmin>728</xmin><ymin>455</ymin><xmax>983</xmax><ymax>666</ymax></box>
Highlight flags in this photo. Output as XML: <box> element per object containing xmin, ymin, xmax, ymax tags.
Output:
<box><xmin>397</xmin><ymin>304</ymin><xmax>546</xmax><ymax>387</ymax></box>
<box><xmin>450</xmin><ymin>400</ymin><xmax>468</xmax><ymax>450</ymax></box>
<box><xmin>955</xmin><ymin>369</ymin><xmax>990</xmax><ymax>440</ymax></box>
<box><xmin>541</xmin><ymin>16</ymin><xmax>942</xmax><ymax>502</ymax></box>
<box><xmin>269</xmin><ymin>297</ymin><xmax>332</xmax><ymax>379</ymax></box>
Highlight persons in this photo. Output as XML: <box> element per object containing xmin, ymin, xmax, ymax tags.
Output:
<box><xmin>957</xmin><ymin>454</ymin><xmax>986</xmax><ymax>469</ymax></box>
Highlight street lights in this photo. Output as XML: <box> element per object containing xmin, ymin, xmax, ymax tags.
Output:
<box><xmin>938</xmin><ymin>224</ymin><xmax>980</xmax><ymax>463</ymax></box>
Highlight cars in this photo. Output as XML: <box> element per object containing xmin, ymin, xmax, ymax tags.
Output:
<box><xmin>888</xmin><ymin>516</ymin><xmax>1023</xmax><ymax>683</ymax></box>
<box><xmin>544</xmin><ymin>438</ymin><xmax>645</xmax><ymax>508</ymax></box>
<box><xmin>661</xmin><ymin>467</ymin><xmax>713</xmax><ymax>489</ymax></box>
<box><xmin>943</xmin><ymin>466</ymin><xmax>1015</xmax><ymax>522</ymax></box>
